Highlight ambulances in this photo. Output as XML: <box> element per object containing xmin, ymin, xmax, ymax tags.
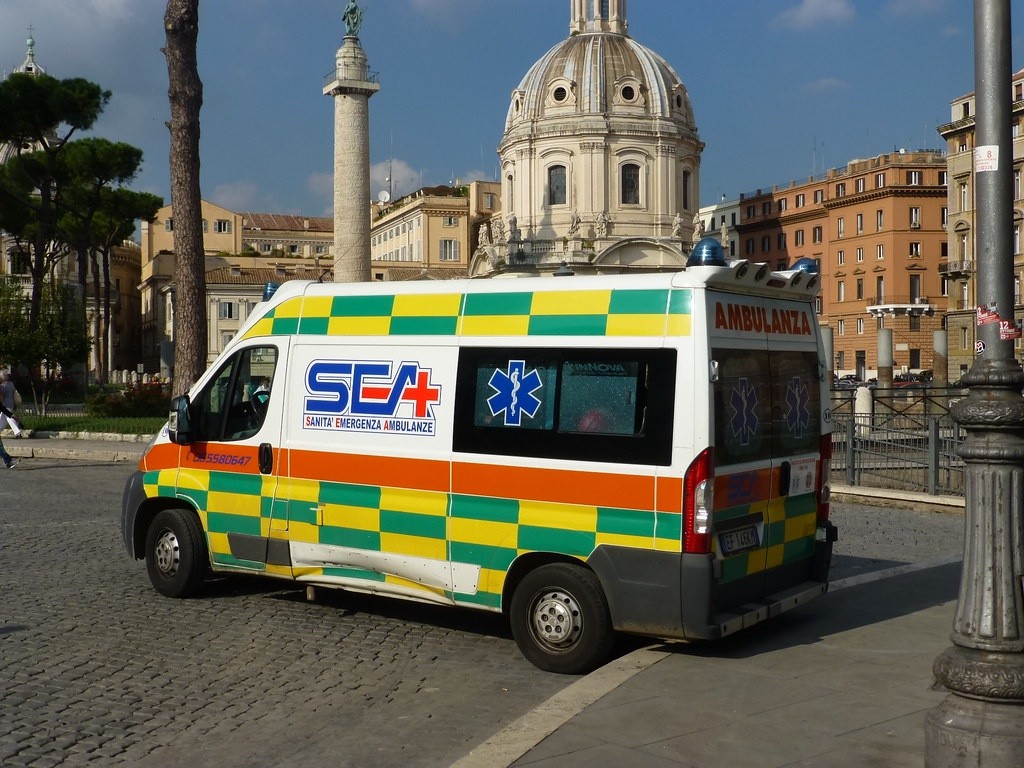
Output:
<box><xmin>118</xmin><ymin>237</ymin><xmax>842</xmax><ymax>674</ymax></box>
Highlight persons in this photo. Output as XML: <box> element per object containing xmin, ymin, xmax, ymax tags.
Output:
<box><xmin>253</xmin><ymin>379</ymin><xmax>270</xmax><ymax>398</ymax></box>
<box><xmin>478</xmin><ymin>216</ymin><xmax>506</xmax><ymax>244</ymax></box>
<box><xmin>0</xmin><ymin>370</ymin><xmax>22</xmax><ymax>440</ymax></box>
<box><xmin>673</xmin><ymin>213</ymin><xmax>684</xmax><ymax>237</ymax></box>
<box><xmin>509</xmin><ymin>212</ymin><xmax>518</xmax><ymax>241</ymax></box>
<box><xmin>571</xmin><ymin>208</ymin><xmax>580</xmax><ymax>233</ymax></box>
<box><xmin>0</xmin><ymin>402</ymin><xmax>18</xmax><ymax>469</ymax></box>
<box><xmin>720</xmin><ymin>222</ymin><xmax>729</xmax><ymax>247</ymax></box>
<box><xmin>596</xmin><ymin>210</ymin><xmax>610</xmax><ymax>236</ymax></box>
<box><xmin>693</xmin><ymin>213</ymin><xmax>702</xmax><ymax>238</ymax></box>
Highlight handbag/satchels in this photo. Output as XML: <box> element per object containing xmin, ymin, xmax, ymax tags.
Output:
<box><xmin>12</xmin><ymin>384</ymin><xmax>22</xmax><ymax>408</ymax></box>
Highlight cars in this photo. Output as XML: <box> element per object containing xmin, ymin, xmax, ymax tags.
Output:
<box><xmin>830</xmin><ymin>371</ymin><xmax>932</xmax><ymax>398</ymax></box>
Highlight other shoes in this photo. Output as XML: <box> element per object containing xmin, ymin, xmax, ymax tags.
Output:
<box><xmin>16</xmin><ymin>433</ymin><xmax>21</xmax><ymax>439</ymax></box>
<box><xmin>7</xmin><ymin>460</ymin><xmax>19</xmax><ymax>469</ymax></box>
